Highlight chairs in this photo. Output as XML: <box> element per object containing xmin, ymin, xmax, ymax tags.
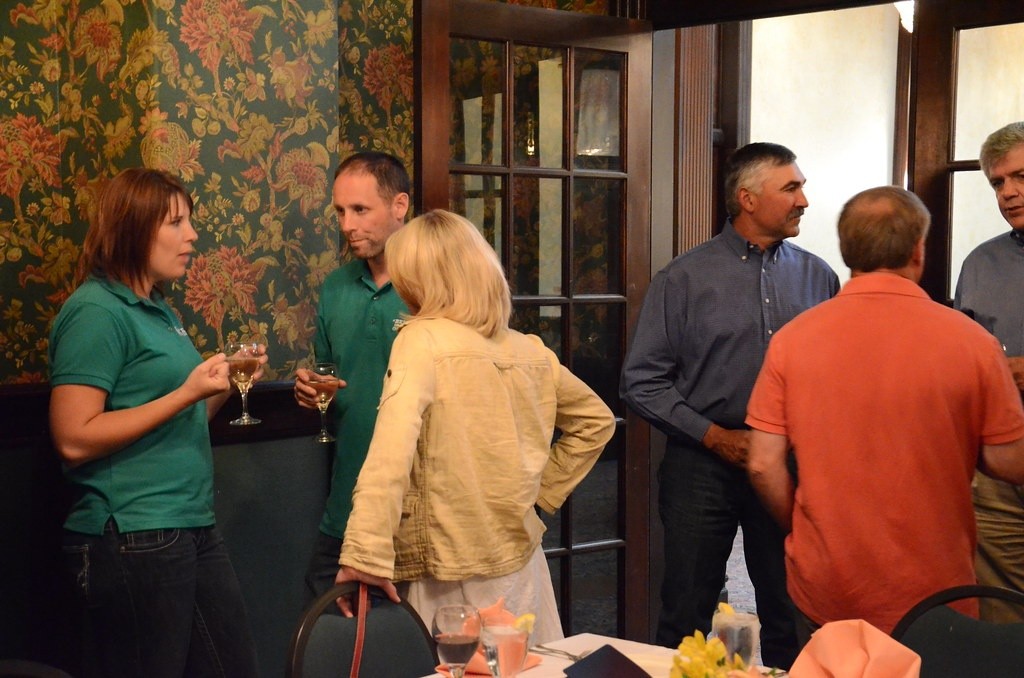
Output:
<box><xmin>290</xmin><ymin>578</ymin><xmax>443</xmax><ymax>678</ymax></box>
<box><xmin>886</xmin><ymin>581</ymin><xmax>1024</xmax><ymax>678</ymax></box>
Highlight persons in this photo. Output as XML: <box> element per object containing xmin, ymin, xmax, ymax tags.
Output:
<box><xmin>42</xmin><ymin>166</ymin><xmax>262</xmax><ymax>678</ymax></box>
<box><xmin>616</xmin><ymin>142</ymin><xmax>841</xmax><ymax>671</ymax></box>
<box><xmin>952</xmin><ymin>121</ymin><xmax>1023</xmax><ymax>626</ymax></box>
<box><xmin>331</xmin><ymin>208</ymin><xmax>617</xmax><ymax>665</ymax></box>
<box><xmin>292</xmin><ymin>149</ymin><xmax>416</xmax><ymax>615</ymax></box>
<box><xmin>743</xmin><ymin>186</ymin><xmax>1023</xmax><ymax>650</ymax></box>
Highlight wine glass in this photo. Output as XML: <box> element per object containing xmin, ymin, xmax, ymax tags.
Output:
<box><xmin>305</xmin><ymin>362</ymin><xmax>337</xmax><ymax>444</ymax></box>
<box><xmin>432</xmin><ymin>604</ymin><xmax>483</xmax><ymax>678</ymax></box>
<box><xmin>223</xmin><ymin>341</ymin><xmax>263</xmax><ymax>425</ymax></box>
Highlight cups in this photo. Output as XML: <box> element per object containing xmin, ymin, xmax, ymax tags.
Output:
<box><xmin>478</xmin><ymin>622</ymin><xmax>530</xmax><ymax>677</ymax></box>
<box><xmin>711</xmin><ymin>608</ymin><xmax>760</xmax><ymax>671</ymax></box>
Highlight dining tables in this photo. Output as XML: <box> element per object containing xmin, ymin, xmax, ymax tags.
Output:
<box><xmin>445</xmin><ymin>633</ymin><xmax>790</xmax><ymax>678</ymax></box>
